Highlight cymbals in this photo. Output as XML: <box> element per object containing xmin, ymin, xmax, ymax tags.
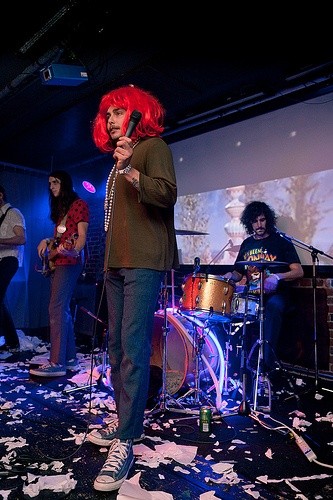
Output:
<box><xmin>175</xmin><ymin>230</ymin><xmax>211</xmax><ymax>236</ymax></box>
<box><xmin>235</xmin><ymin>258</ymin><xmax>289</xmax><ymax>265</ymax></box>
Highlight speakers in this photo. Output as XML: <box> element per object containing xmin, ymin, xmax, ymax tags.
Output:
<box><xmin>70</xmin><ymin>283</ymin><xmax>108</xmax><ymax>337</ymax></box>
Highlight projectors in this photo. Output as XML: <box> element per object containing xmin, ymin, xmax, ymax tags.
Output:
<box><xmin>40</xmin><ymin>64</ymin><xmax>88</xmax><ymax>85</ymax></box>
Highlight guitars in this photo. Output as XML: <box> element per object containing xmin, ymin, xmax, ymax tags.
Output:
<box><xmin>38</xmin><ymin>233</ymin><xmax>80</xmax><ymax>279</ymax></box>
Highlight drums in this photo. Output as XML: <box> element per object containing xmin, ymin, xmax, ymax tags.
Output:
<box><xmin>147</xmin><ymin>308</ymin><xmax>221</xmax><ymax>401</ymax></box>
<box><xmin>231</xmin><ymin>292</ymin><xmax>261</xmax><ymax>321</ymax></box>
<box><xmin>180</xmin><ymin>273</ymin><xmax>235</xmax><ymax>324</ymax></box>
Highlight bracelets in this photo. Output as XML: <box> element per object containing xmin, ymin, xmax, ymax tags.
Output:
<box><xmin>271</xmin><ymin>274</ymin><xmax>280</xmax><ymax>280</ymax></box>
<box><xmin>119</xmin><ymin>164</ymin><xmax>130</xmax><ymax>175</ymax></box>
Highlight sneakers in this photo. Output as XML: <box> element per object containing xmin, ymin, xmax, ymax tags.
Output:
<box><xmin>93</xmin><ymin>437</ymin><xmax>135</xmax><ymax>491</ymax></box>
<box><xmin>88</xmin><ymin>419</ymin><xmax>146</xmax><ymax>446</ymax></box>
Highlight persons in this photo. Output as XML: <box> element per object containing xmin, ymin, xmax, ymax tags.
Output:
<box><xmin>88</xmin><ymin>86</ymin><xmax>180</xmax><ymax>491</ymax></box>
<box><xmin>223</xmin><ymin>202</ymin><xmax>304</xmax><ymax>365</ymax></box>
<box><xmin>30</xmin><ymin>171</ymin><xmax>89</xmax><ymax>376</ymax></box>
<box><xmin>0</xmin><ymin>185</ymin><xmax>26</xmax><ymax>354</ymax></box>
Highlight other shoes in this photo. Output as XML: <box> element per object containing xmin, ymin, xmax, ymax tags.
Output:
<box><xmin>0</xmin><ymin>344</ymin><xmax>21</xmax><ymax>353</ymax></box>
<box><xmin>29</xmin><ymin>361</ymin><xmax>67</xmax><ymax>377</ymax></box>
<box><xmin>66</xmin><ymin>358</ymin><xmax>82</xmax><ymax>372</ymax></box>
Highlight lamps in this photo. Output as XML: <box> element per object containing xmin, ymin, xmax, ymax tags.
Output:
<box><xmin>40</xmin><ymin>64</ymin><xmax>90</xmax><ymax>85</ymax></box>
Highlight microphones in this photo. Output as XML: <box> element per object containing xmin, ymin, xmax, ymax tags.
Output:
<box><xmin>272</xmin><ymin>226</ymin><xmax>282</xmax><ymax>236</ymax></box>
<box><xmin>125</xmin><ymin>110</ymin><xmax>142</xmax><ymax>137</ymax></box>
<box><xmin>194</xmin><ymin>257</ymin><xmax>200</xmax><ymax>271</ymax></box>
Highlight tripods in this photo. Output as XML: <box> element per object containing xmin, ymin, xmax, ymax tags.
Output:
<box><xmin>148</xmin><ymin>233</ymin><xmax>333</xmax><ymax>435</ymax></box>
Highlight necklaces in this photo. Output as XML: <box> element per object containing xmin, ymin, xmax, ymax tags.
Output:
<box><xmin>104</xmin><ymin>164</ymin><xmax>115</xmax><ymax>231</ymax></box>
